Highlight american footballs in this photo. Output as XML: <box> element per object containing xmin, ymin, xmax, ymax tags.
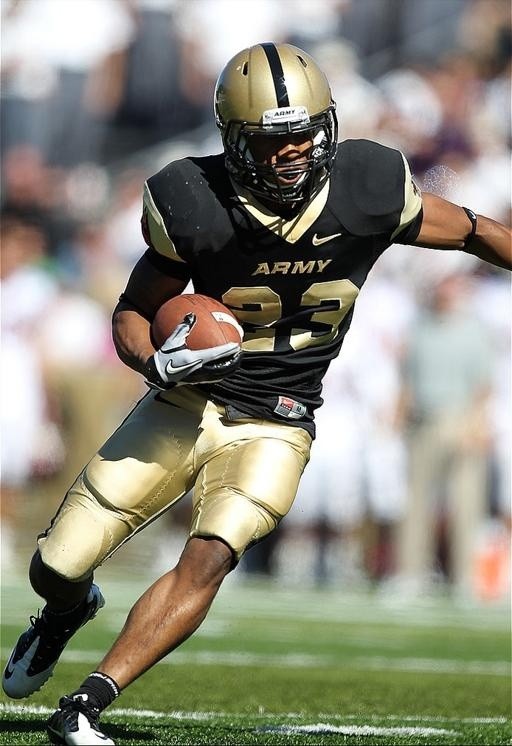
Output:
<box><xmin>153</xmin><ymin>294</ymin><xmax>244</xmax><ymax>366</ymax></box>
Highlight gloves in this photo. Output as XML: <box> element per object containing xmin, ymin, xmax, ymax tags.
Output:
<box><xmin>144</xmin><ymin>311</ymin><xmax>243</xmax><ymax>391</ymax></box>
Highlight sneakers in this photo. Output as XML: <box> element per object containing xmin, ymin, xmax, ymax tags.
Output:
<box><xmin>2</xmin><ymin>584</ymin><xmax>104</xmax><ymax>701</ymax></box>
<box><xmin>45</xmin><ymin>690</ymin><xmax>115</xmax><ymax>744</ymax></box>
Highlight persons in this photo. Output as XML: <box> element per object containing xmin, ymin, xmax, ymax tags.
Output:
<box><xmin>0</xmin><ymin>41</ymin><xmax>511</xmax><ymax>746</ymax></box>
<box><xmin>0</xmin><ymin>0</ymin><xmax>510</xmax><ymax>605</ymax></box>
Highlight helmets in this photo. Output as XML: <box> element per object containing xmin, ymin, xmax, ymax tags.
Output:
<box><xmin>213</xmin><ymin>41</ymin><xmax>340</xmax><ymax>212</ymax></box>
